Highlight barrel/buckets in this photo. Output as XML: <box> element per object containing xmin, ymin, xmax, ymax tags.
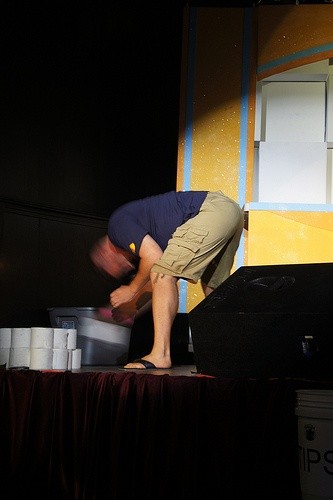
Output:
<box><xmin>45</xmin><ymin>307</ymin><xmax>134</xmax><ymax>366</ymax></box>
<box><xmin>294</xmin><ymin>390</ymin><xmax>333</xmax><ymax>500</ymax></box>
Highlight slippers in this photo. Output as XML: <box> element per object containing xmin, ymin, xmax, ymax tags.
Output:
<box><xmin>124</xmin><ymin>359</ymin><xmax>175</xmax><ymax>371</ymax></box>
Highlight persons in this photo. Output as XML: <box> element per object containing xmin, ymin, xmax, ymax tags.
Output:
<box><xmin>87</xmin><ymin>191</ymin><xmax>247</xmax><ymax>372</ymax></box>
